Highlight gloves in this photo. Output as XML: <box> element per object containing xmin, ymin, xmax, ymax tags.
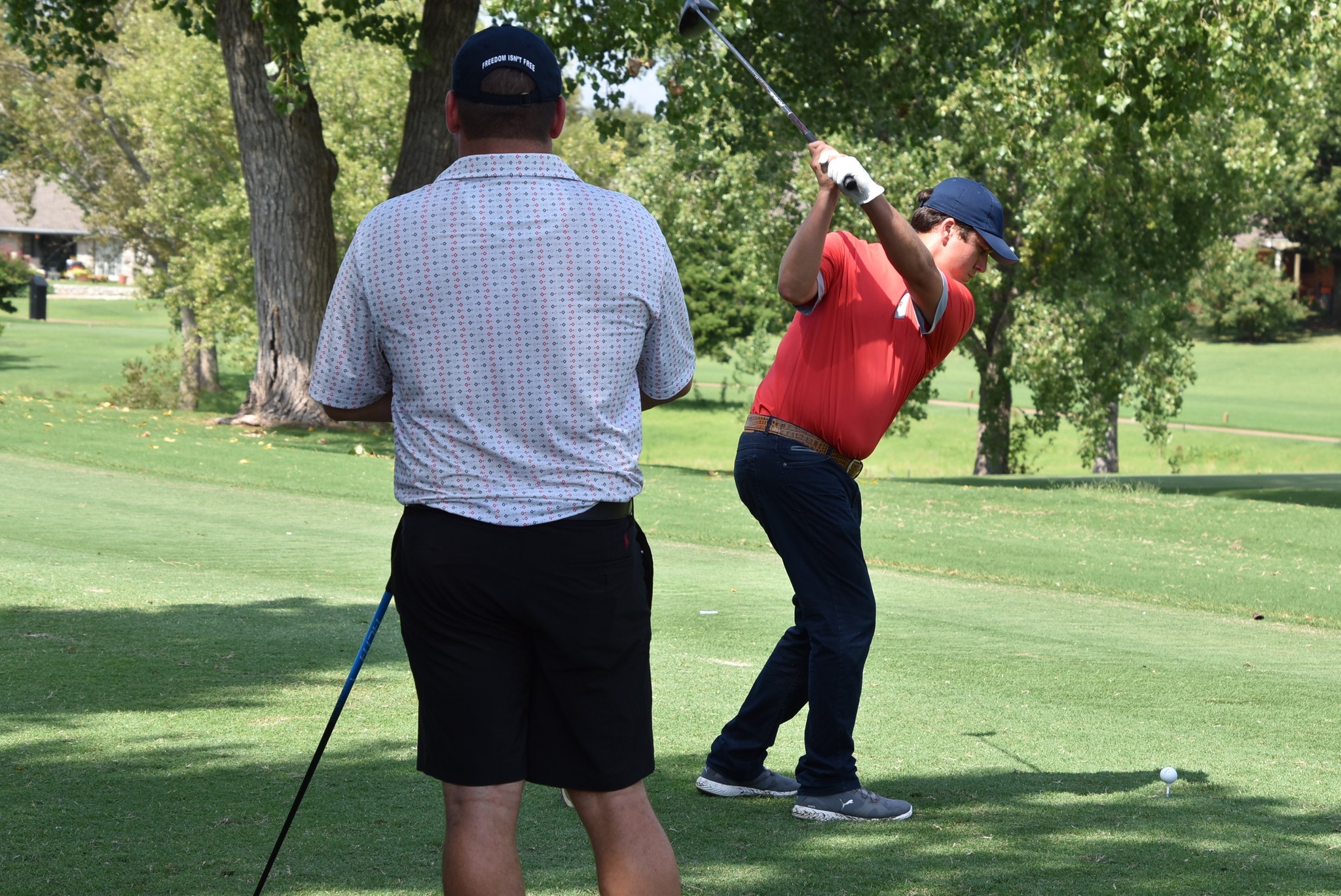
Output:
<box><xmin>818</xmin><ymin>150</ymin><xmax>885</xmax><ymax>205</ymax></box>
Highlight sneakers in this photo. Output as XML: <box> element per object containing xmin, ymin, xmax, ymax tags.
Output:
<box><xmin>791</xmin><ymin>787</ymin><xmax>912</xmax><ymax>823</ymax></box>
<box><xmin>696</xmin><ymin>765</ymin><xmax>802</xmax><ymax>797</ymax></box>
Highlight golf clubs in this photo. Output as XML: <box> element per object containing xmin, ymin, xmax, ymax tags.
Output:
<box><xmin>248</xmin><ymin>575</ymin><xmax>396</xmax><ymax>896</ymax></box>
<box><xmin>675</xmin><ymin>1</ymin><xmax>859</xmax><ymax>194</ymax></box>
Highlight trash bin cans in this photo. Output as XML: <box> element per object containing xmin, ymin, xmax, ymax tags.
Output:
<box><xmin>29</xmin><ymin>278</ymin><xmax>47</xmax><ymax>320</ymax></box>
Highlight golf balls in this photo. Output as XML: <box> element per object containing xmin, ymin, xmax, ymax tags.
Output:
<box><xmin>1159</xmin><ymin>767</ymin><xmax>1179</xmax><ymax>784</ymax></box>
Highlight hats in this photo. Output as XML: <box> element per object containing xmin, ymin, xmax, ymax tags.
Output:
<box><xmin>450</xmin><ymin>24</ymin><xmax>562</xmax><ymax>105</ymax></box>
<box><xmin>919</xmin><ymin>177</ymin><xmax>1019</xmax><ymax>265</ymax></box>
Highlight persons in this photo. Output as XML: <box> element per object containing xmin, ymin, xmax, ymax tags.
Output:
<box><xmin>308</xmin><ymin>23</ymin><xmax>697</xmax><ymax>895</ymax></box>
<box><xmin>695</xmin><ymin>141</ymin><xmax>1020</xmax><ymax>822</ymax></box>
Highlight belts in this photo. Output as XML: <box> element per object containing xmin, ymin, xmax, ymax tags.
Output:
<box><xmin>744</xmin><ymin>414</ymin><xmax>863</xmax><ymax>479</ymax></box>
<box><xmin>410</xmin><ymin>500</ymin><xmax>631</xmax><ymax>520</ymax></box>
